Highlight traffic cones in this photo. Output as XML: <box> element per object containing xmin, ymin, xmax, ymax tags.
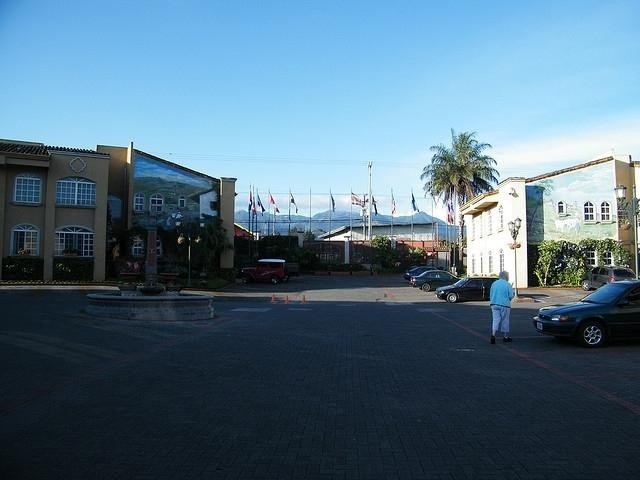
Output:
<box><xmin>270</xmin><ymin>293</ymin><xmax>278</xmax><ymax>304</ymax></box>
<box><xmin>283</xmin><ymin>294</ymin><xmax>290</xmax><ymax>304</ymax></box>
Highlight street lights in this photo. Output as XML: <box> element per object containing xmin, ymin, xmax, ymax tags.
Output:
<box><xmin>508</xmin><ymin>216</ymin><xmax>524</xmax><ymax>299</ymax></box>
<box><xmin>614</xmin><ymin>183</ymin><xmax>640</xmax><ymax>280</ymax></box>
<box><xmin>176</xmin><ymin>233</ymin><xmax>203</xmax><ymax>283</ymax></box>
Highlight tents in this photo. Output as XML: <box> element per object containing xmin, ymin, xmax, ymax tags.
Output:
<box><xmin>331</xmin><ymin>194</ymin><xmax>336</xmax><ymax>212</ymax></box>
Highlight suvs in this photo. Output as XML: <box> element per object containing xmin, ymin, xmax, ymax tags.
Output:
<box><xmin>239</xmin><ymin>258</ymin><xmax>289</xmax><ymax>285</ymax></box>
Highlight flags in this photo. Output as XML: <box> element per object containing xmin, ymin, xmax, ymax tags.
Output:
<box><xmin>257</xmin><ymin>196</ymin><xmax>265</xmax><ymax>216</ymax></box>
<box><xmin>253</xmin><ymin>198</ymin><xmax>256</xmax><ymax>219</ymax></box>
<box><xmin>372</xmin><ymin>196</ymin><xmax>378</xmax><ymax>216</ymax></box>
<box><xmin>351</xmin><ymin>193</ymin><xmax>364</xmax><ymax>207</ymax></box>
<box><xmin>269</xmin><ymin>194</ymin><xmax>275</xmax><ymax>204</ymax></box>
<box><xmin>447</xmin><ymin>203</ymin><xmax>451</xmax><ymax>224</ymax></box>
<box><xmin>411</xmin><ymin>194</ymin><xmax>422</xmax><ymax>213</ymax></box>
<box><xmin>392</xmin><ymin>194</ymin><xmax>395</xmax><ymax>214</ymax></box>
<box><xmin>290</xmin><ymin>194</ymin><xmax>298</xmax><ymax>213</ymax></box>
<box><xmin>249</xmin><ymin>192</ymin><xmax>252</xmax><ymax>211</ymax></box>
<box><xmin>275</xmin><ymin>208</ymin><xmax>280</xmax><ymax>214</ymax></box>
<box><xmin>451</xmin><ymin>205</ymin><xmax>455</xmax><ymax>224</ymax></box>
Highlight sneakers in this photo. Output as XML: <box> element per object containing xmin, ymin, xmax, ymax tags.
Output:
<box><xmin>490</xmin><ymin>335</ymin><xmax>495</xmax><ymax>344</ymax></box>
<box><xmin>504</xmin><ymin>337</ymin><xmax>513</xmax><ymax>342</ymax></box>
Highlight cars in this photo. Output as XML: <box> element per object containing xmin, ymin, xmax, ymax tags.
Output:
<box><xmin>579</xmin><ymin>265</ymin><xmax>640</xmax><ymax>292</ymax></box>
<box><xmin>434</xmin><ymin>276</ymin><xmax>500</xmax><ymax>304</ymax></box>
<box><xmin>401</xmin><ymin>264</ymin><xmax>461</xmax><ymax>292</ymax></box>
<box><xmin>531</xmin><ymin>278</ymin><xmax>640</xmax><ymax>349</ymax></box>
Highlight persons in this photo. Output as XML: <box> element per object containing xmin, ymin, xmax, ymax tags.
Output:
<box><xmin>489</xmin><ymin>270</ymin><xmax>515</xmax><ymax>344</ymax></box>
